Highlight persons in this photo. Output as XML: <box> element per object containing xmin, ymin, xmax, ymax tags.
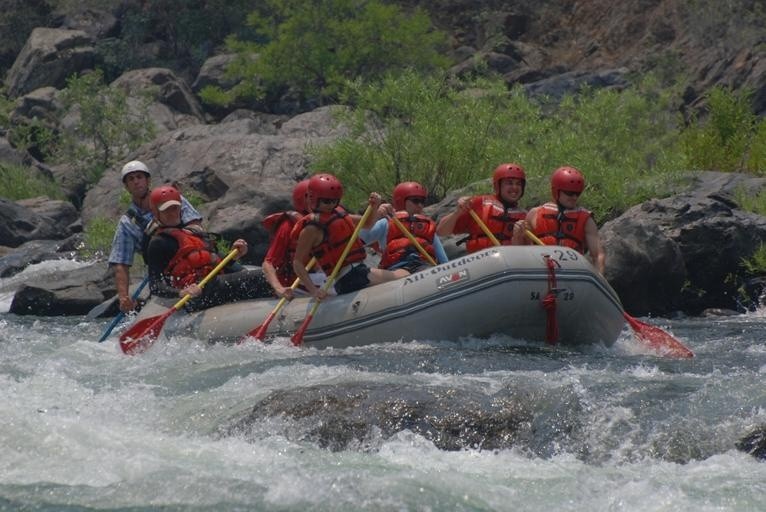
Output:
<box><xmin>141</xmin><ymin>186</ymin><xmax>276</xmax><ymax>314</ymax></box>
<box><xmin>357</xmin><ymin>181</ymin><xmax>449</xmax><ymax>275</ymax></box>
<box><xmin>108</xmin><ymin>160</ymin><xmax>207</xmax><ymax>314</ymax></box>
<box><xmin>435</xmin><ymin>163</ymin><xmax>529</xmax><ymax>254</ymax></box>
<box><xmin>511</xmin><ymin>166</ymin><xmax>605</xmax><ymax>276</ymax></box>
<box><xmin>290</xmin><ymin>173</ymin><xmax>411</xmax><ymax>302</ymax></box>
<box><xmin>261</xmin><ymin>178</ymin><xmax>338</xmax><ymax>302</ymax></box>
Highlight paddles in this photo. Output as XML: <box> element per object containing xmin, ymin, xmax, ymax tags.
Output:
<box><xmin>120</xmin><ymin>248</ymin><xmax>239</xmax><ymax>355</ymax></box>
<box><xmin>525</xmin><ymin>230</ymin><xmax>695</xmax><ymax>357</ymax></box>
<box><xmin>290</xmin><ymin>202</ymin><xmax>376</xmax><ymax>347</ymax></box>
<box><xmin>236</xmin><ymin>256</ymin><xmax>317</xmax><ymax>345</ymax></box>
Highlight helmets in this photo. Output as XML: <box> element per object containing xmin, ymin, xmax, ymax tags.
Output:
<box><xmin>550</xmin><ymin>167</ymin><xmax>585</xmax><ymax>207</ymax></box>
<box><xmin>293</xmin><ymin>173</ymin><xmax>343</xmax><ymax>215</ymax></box>
<box><xmin>392</xmin><ymin>181</ymin><xmax>425</xmax><ymax>213</ymax></box>
<box><xmin>492</xmin><ymin>163</ymin><xmax>525</xmax><ymax>203</ymax></box>
<box><xmin>148</xmin><ymin>186</ymin><xmax>182</xmax><ymax>218</ymax></box>
<box><xmin>121</xmin><ymin>160</ymin><xmax>151</xmax><ymax>182</ymax></box>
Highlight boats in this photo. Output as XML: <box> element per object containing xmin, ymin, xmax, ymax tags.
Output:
<box><xmin>134</xmin><ymin>244</ymin><xmax>624</xmax><ymax>354</ymax></box>
